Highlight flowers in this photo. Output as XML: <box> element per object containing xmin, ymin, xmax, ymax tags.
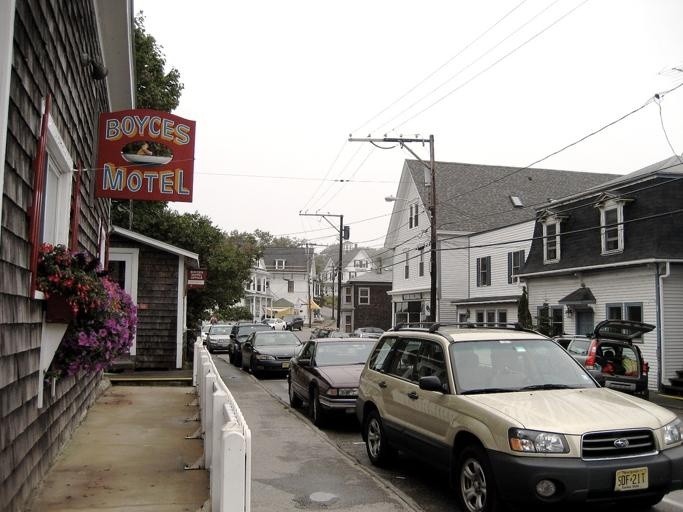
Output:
<box><xmin>36</xmin><ymin>238</ymin><xmax>137</xmax><ymax>384</ymax></box>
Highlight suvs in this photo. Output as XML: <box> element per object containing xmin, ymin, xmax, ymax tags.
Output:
<box><xmin>550</xmin><ymin>318</ymin><xmax>656</xmax><ymax>398</ymax></box>
<box><xmin>356</xmin><ymin>321</ymin><xmax>682</xmax><ymax>510</ymax></box>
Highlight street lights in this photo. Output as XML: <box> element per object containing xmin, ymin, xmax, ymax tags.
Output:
<box><xmin>385</xmin><ymin>194</ymin><xmax>436</xmax><ymax>324</ymax></box>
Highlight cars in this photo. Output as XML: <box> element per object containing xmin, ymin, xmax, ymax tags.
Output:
<box><xmin>203</xmin><ymin>317</ymin><xmax>304</xmax><ymax>377</ymax></box>
<box><xmin>289</xmin><ymin>326</ymin><xmax>386</xmax><ymax>426</ymax></box>
